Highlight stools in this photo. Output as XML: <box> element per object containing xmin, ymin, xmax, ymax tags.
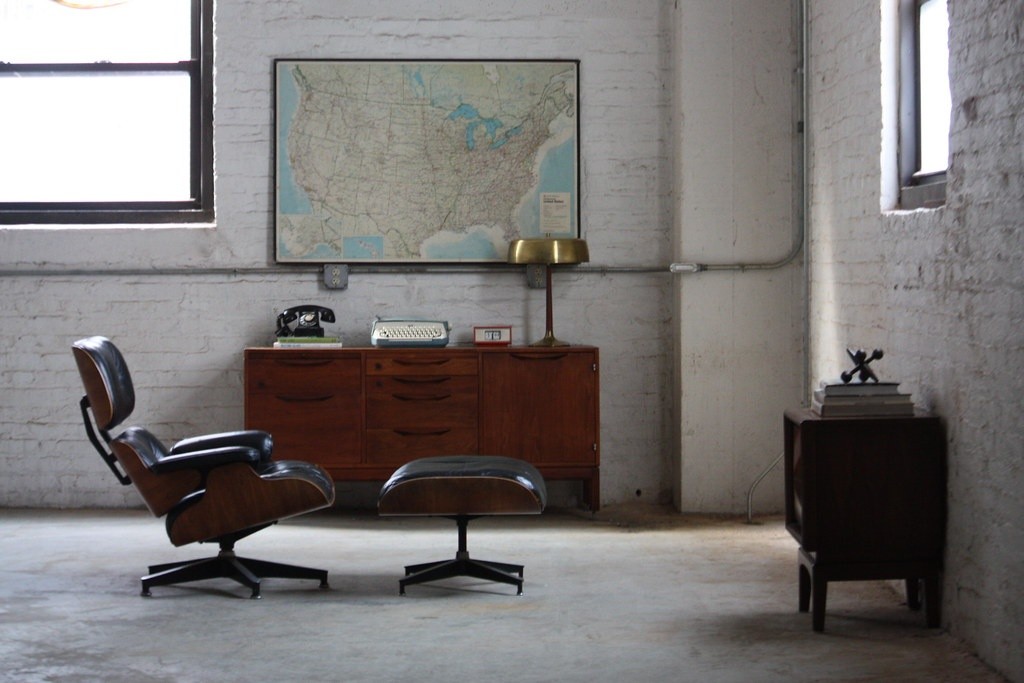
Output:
<box><xmin>377</xmin><ymin>455</ymin><xmax>548</xmax><ymax>596</ymax></box>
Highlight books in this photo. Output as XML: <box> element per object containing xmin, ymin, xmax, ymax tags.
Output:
<box><xmin>273</xmin><ymin>336</ymin><xmax>343</xmax><ymax>349</ymax></box>
<box><xmin>812</xmin><ymin>378</ymin><xmax>915</xmax><ymax>417</ymax></box>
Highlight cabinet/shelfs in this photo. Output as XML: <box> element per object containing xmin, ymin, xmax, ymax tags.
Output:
<box><xmin>783</xmin><ymin>405</ymin><xmax>949</xmax><ymax>633</ymax></box>
<box><xmin>243</xmin><ymin>343</ymin><xmax>601</xmax><ymax>517</ymax></box>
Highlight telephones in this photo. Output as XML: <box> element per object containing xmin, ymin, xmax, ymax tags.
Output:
<box><xmin>274</xmin><ymin>304</ymin><xmax>336</xmax><ymax>338</ymax></box>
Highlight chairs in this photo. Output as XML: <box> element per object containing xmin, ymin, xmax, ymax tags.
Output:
<box><xmin>70</xmin><ymin>335</ymin><xmax>335</xmax><ymax>601</ymax></box>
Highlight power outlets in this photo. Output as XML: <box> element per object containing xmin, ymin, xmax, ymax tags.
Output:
<box><xmin>526</xmin><ymin>264</ymin><xmax>547</xmax><ymax>289</ymax></box>
<box><xmin>323</xmin><ymin>264</ymin><xmax>349</xmax><ymax>289</ymax></box>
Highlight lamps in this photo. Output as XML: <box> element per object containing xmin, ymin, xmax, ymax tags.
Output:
<box><xmin>507</xmin><ymin>233</ymin><xmax>591</xmax><ymax>347</ymax></box>
